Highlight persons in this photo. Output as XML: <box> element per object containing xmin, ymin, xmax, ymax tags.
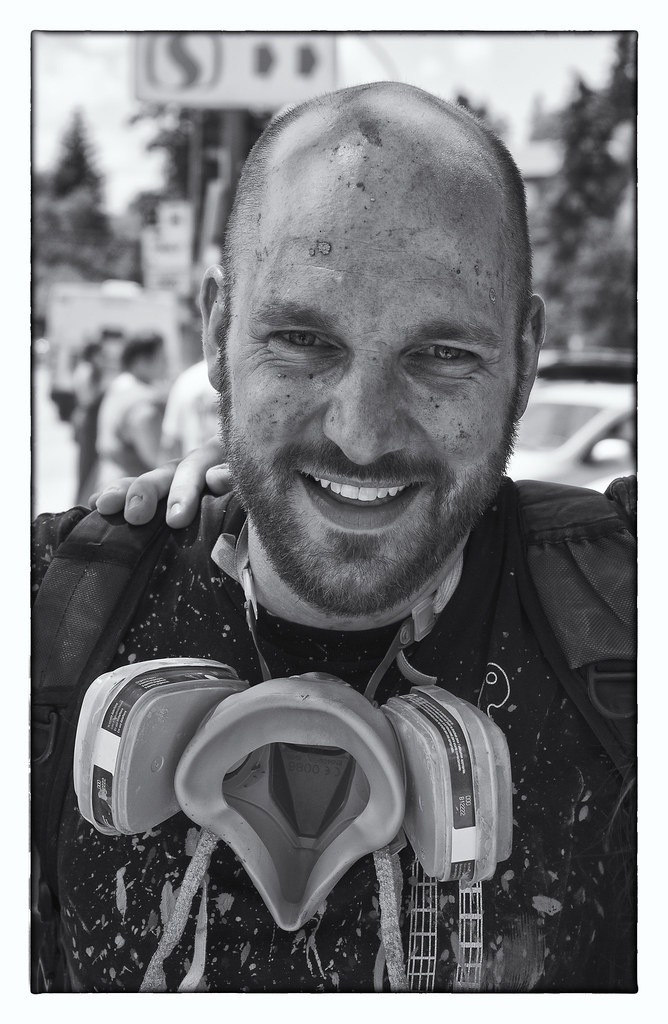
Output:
<box><xmin>34</xmin><ymin>315</ymin><xmax>235</xmax><ymax>529</ymax></box>
<box><xmin>31</xmin><ymin>82</ymin><xmax>638</xmax><ymax>993</ymax></box>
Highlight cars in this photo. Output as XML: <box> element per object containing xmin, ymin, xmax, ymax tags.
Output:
<box><xmin>503</xmin><ymin>377</ymin><xmax>636</xmax><ymax>494</ymax></box>
